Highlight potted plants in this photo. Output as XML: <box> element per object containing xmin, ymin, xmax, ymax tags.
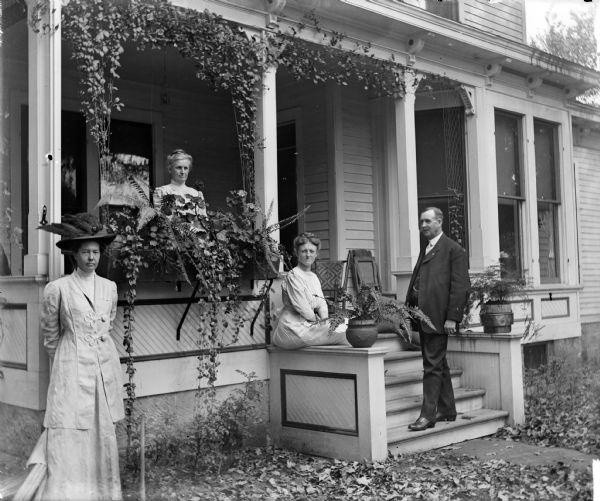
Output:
<box><xmin>326</xmin><ymin>281</ymin><xmax>436</xmax><ymax>349</ymax></box>
<box><xmin>464</xmin><ymin>255</ymin><xmax>543</xmax><ymax>337</ymax></box>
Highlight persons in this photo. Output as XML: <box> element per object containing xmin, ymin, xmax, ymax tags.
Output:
<box><xmin>12</xmin><ymin>234</ymin><xmax>125</xmax><ymax>501</ymax></box>
<box><xmin>400</xmin><ymin>206</ymin><xmax>470</xmax><ymax>432</ymax></box>
<box><xmin>153</xmin><ymin>150</ymin><xmax>207</xmax><ymax>228</ymax></box>
<box><xmin>272</xmin><ymin>231</ymin><xmax>352</xmax><ymax>351</ymax></box>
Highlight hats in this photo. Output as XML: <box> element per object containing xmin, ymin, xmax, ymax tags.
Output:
<box><xmin>55</xmin><ymin>213</ymin><xmax>117</xmax><ymax>249</ymax></box>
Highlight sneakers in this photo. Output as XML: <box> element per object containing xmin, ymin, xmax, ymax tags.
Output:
<box><xmin>435</xmin><ymin>411</ymin><xmax>458</xmax><ymax>422</ymax></box>
<box><xmin>408</xmin><ymin>415</ymin><xmax>436</xmax><ymax>430</ymax></box>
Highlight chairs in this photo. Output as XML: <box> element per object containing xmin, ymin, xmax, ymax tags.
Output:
<box><xmin>312</xmin><ymin>249</ymin><xmax>397</xmax><ymax>314</ymax></box>
